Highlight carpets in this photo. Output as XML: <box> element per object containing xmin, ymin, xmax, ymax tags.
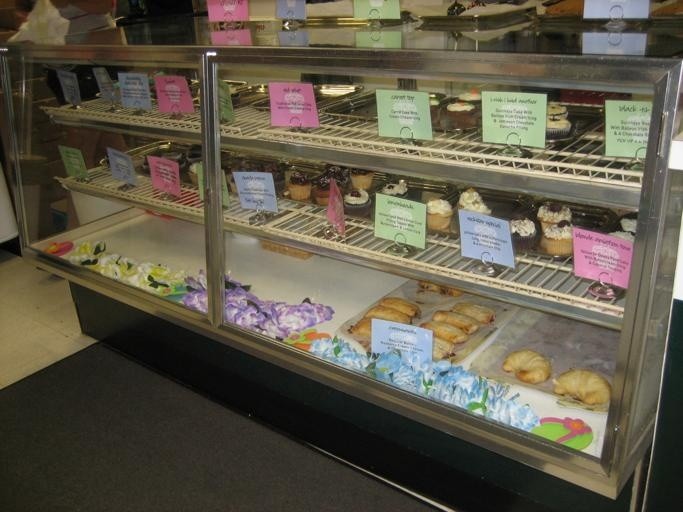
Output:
<box><xmin>4</xmin><ymin>297</ymin><xmax>683</xmax><ymax>510</ymax></box>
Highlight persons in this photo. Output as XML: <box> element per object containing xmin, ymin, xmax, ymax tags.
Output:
<box><xmin>5</xmin><ymin>0</ymin><xmax>133</xmax><ymax>281</ymax></box>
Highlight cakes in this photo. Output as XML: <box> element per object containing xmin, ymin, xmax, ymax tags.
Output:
<box><xmin>429</xmin><ymin>88</ymin><xmax>572</xmax><ymax>138</ymax></box>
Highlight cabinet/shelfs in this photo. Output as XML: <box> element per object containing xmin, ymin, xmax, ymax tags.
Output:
<box><xmin>0</xmin><ymin>47</ymin><xmax>683</xmax><ymax>512</ymax></box>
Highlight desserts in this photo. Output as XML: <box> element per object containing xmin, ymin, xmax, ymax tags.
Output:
<box><xmin>288</xmin><ymin>167</ymin><xmax>636</xmax><ymax>257</ymax></box>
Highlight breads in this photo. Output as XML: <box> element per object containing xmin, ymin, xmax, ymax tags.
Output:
<box><xmin>346</xmin><ymin>279</ymin><xmax>494</xmax><ymax>361</ymax></box>
<box><xmin>553</xmin><ymin>369</ymin><xmax>613</xmax><ymax>406</ymax></box>
<box><xmin>501</xmin><ymin>348</ymin><xmax>549</xmax><ymax>384</ymax></box>
